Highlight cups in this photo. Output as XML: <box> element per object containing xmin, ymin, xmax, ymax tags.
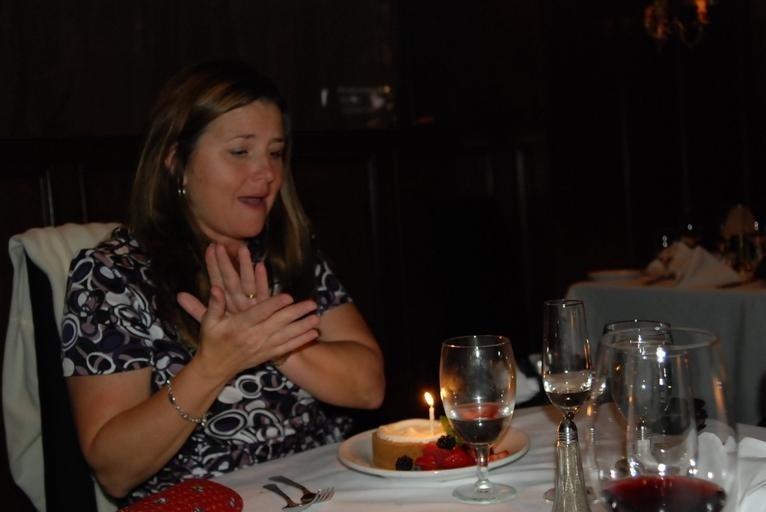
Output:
<box><xmin>585</xmin><ymin>329</ymin><xmax>737</xmax><ymax>512</ymax></box>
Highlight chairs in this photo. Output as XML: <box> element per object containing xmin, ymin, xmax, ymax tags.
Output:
<box><xmin>21</xmin><ymin>236</ymin><xmax>100</xmax><ymax>512</ymax></box>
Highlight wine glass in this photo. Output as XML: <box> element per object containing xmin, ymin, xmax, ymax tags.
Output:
<box><xmin>602</xmin><ymin>321</ymin><xmax>670</xmax><ymax>479</ymax></box>
<box><xmin>540</xmin><ymin>299</ymin><xmax>600</xmax><ymax>504</ymax></box>
<box><xmin>439</xmin><ymin>335</ymin><xmax>515</xmax><ymax>505</ymax></box>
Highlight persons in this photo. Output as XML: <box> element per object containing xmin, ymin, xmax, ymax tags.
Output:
<box><xmin>55</xmin><ymin>55</ymin><xmax>390</xmax><ymax>512</ymax></box>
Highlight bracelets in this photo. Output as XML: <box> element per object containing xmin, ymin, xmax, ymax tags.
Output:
<box><xmin>164</xmin><ymin>377</ymin><xmax>208</xmax><ymax>428</ymax></box>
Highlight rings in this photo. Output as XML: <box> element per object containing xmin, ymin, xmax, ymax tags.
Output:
<box><xmin>245</xmin><ymin>293</ymin><xmax>257</xmax><ymax>299</ymax></box>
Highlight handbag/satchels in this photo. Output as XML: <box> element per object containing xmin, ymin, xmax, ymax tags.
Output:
<box><xmin>115</xmin><ymin>476</ymin><xmax>243</xmax><ymax>512</ymax></box>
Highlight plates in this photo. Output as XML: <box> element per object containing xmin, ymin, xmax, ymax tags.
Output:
<box><xmin>339</xmin><ymin>418</ymin><xmax>530</xmax><ymax>482</ymax></box>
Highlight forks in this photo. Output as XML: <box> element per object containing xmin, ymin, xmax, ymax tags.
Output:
<box><xmin>265</xmin><ymin>473</ymin><xmax>334</xmax><ymax>510</ymax></box>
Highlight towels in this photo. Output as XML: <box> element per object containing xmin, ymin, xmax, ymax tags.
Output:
<box><xmin>673</xmin><ymin>247</ymin><xmax>739</xmax><ymax>296</ymax></box>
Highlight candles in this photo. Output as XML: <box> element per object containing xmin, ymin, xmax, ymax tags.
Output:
<box><xmin>429</xmin><ymin>406</ymin><xmax>435</xmax><ymax>436</ymax></box>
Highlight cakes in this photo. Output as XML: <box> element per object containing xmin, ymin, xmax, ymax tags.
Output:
<box><xmin>372</xmin><ymin>418</ymin><xmax>448</xmax><ymax>470</ymax></box>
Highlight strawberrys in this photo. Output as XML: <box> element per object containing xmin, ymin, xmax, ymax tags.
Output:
<box><xmin>415</xmin><ymin>441</ymin><xmax>476</xmax><ymax>470</ymax></box>
